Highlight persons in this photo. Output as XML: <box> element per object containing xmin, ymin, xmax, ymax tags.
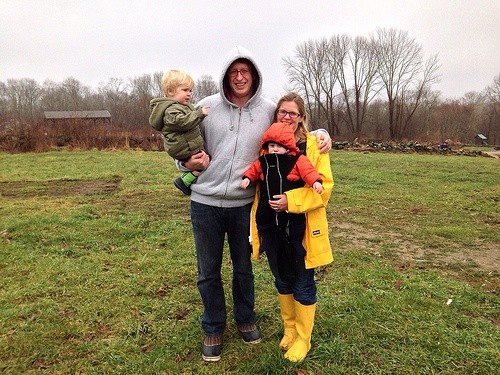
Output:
<box><xmin>440</xmin><ymin>142</ymin><xmax>448</xmax><ymax>150</ymax></box>
<box><xmin>172</xmin><ymin>44</ymin><xmax>333</xmax><ymax>361</ymax></box>
<box><xmin>239</xmin><ymin>123</ymin><xmax>324</xmax><ymax>256</ymax></box>
<box><xmin>477</xmin><ymin>128</ymin><xmax>488</xmax><ymax>145</ymax></box>
<box><xmin>148</xmin><ymin>68</ymin><xmax>212</xmax><ymax>196</ymax></box>
<box><xmin>248</xmin><ymin>92</ymin><xmax>335</xmax><ymax>362</ymax></box>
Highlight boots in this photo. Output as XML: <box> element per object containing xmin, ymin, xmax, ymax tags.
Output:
<box><xmin>278</xmin><ymin>290</ymin><xmax>298</xmax><ymax>351</ymax></box>
<box><xmin>283</xmin><ymin>298</ymin><xmax>317</xmax><ymax>363</ymax></box>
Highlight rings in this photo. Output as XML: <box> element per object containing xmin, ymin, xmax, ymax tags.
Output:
<box><xmin>329</xmin><ymin>145</ymin><xmax>331</xmax><ymax>149</ymax></box>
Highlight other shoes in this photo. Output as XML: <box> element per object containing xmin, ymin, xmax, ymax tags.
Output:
<box><xmin>173</xmin><ymin>177</ymin><xmax>191</xmax><ymax>196</ymax></box>
<box><xmin>236</xmin><ymin>322</ymin><xmax>262</xmax><ymax>344</ymax></box>
<box><xmin>202</xmin><ymin>334</ymin><xmax>223</xmax><ymax>362</ymax></box>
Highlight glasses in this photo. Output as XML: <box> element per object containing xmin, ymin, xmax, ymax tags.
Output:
<box><xmin>278</xmin><ymin>109</ymin><xmax>303</xmax><ymax>118</ymax></box>
<box><xmin>227</xmin><ymin>68</ymin><xmax>251</xmax><ymax>77</ymax></box>
